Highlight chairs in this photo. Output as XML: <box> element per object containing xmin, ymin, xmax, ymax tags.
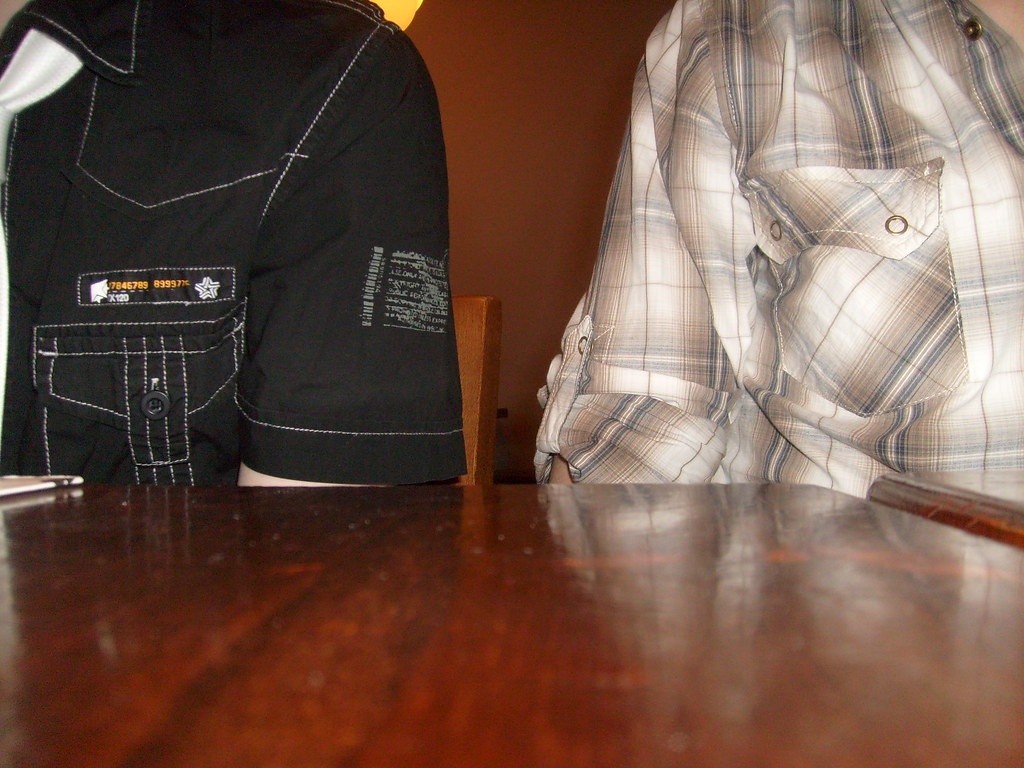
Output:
<box><xmin>451</xmin><ymin>296</ymin><xmax>503</xmax><ymax>485</ymax></box>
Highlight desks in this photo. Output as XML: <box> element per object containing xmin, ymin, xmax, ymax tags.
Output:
<box><xmin>0</xmin><ymin>484</ymin><xmax>1023</xmax><ymax>768</ymax></box>
<box><xmin>867</xmin><ymin>470</ymin><xmax>1024</xmax><ymax>549</ymax></box>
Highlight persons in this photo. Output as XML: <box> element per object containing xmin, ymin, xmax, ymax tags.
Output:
<box><xmin>0</xmin><ymin>0</ymin><xmax>472</xmax><ymax>491</ymax></box>
<box><xmin>530</xmin><ymin>0</ymin><xmax>1024</xmax><ymax>500</ymax></box>
